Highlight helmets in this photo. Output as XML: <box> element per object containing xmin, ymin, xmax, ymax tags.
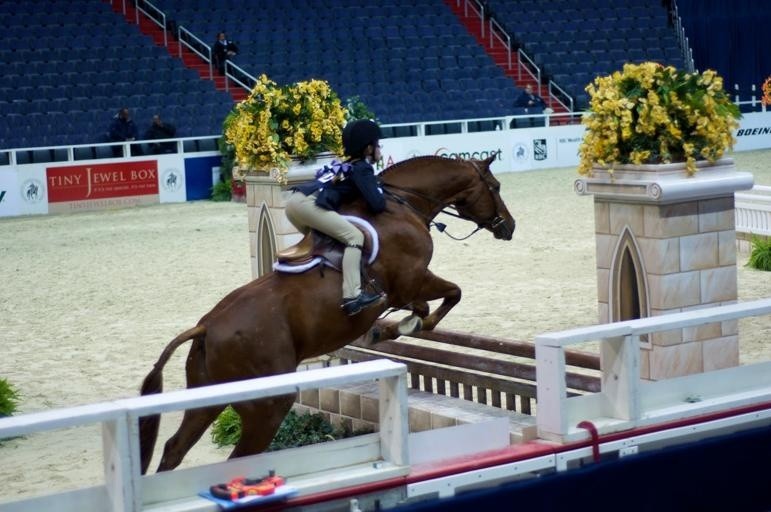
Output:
<box><xmin>343</xmin><ymin>120</ymin><xmax>385</xmax><ymax>156</ymax></box>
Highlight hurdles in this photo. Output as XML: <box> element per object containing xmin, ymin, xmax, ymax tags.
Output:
<box><xmin>232</xmin><ymin>151</ymin><xmax>771</xmax><ymax>444</ymax></box>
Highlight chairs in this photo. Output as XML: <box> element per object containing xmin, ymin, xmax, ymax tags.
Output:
<box><xmin>403</xmin><ymin>424</ymin><xmax>771</xmax><ymax>511</ymax></box>
<box><xmin>0</xmin><ymin>0</ymin><xmax>693</xmax><ymax>165</ymax></box>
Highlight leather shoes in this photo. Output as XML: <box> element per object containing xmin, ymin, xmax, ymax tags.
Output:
<box><xmin>341</xmin><ymin>292</ymin><xmax>381</xmax><ymax>316</ymax></box>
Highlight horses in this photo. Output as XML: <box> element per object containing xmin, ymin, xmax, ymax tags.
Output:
<box><xmin>138</xmin><ymin>152</ymin><xmax>517</xmax><ymax>476</ymax></box>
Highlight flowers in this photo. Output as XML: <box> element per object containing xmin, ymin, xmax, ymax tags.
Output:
<box><xmin>576</xmin><ymin>61</ymin><xmax>743</xmax><ymax>179</ymax></box>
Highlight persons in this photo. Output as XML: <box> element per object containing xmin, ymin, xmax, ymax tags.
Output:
<box><xmin>209</xmin><ymin>32</ymin><xmax>238</xmax><ymax>75</ymax></box>
<box><xmin>146</xmin><ymin>112</ymin><xmax>177</xmax><ymax>155</ymax></box>
<box><xmin>285</xmin><ymin>116</ymin><xmax>389</xmax><ymax>318</ymax></box>
<box><xmin>104</xmin><ymin>104</ymin><xmax>146</xmax><ymax>162</ymax></box>
